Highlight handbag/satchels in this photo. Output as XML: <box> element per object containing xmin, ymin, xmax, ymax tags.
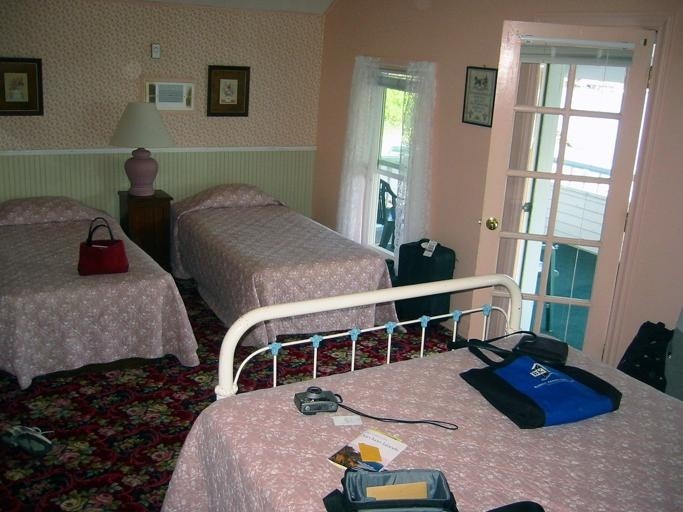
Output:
<box><xmin>78</xmin><ymin>216</ymin><xmax>129</xmax><ymax>275</ymax></box>
<box><xmin>341</xmin><ymin>469</ymin><xmax>458</xmax><ymax>512</ymax></box>
<box><xmin>460</xmin><ymin>335</ymin><xmax>621</xmax><ymax>429</ymax></box>
<box><xmin>618</xmin><ymin>321</ymin><xmax>677</xmax><ymax>392</ymax></box>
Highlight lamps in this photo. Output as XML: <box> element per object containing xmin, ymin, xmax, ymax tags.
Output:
<box><xmin>109</xmin><ymin>102</ymin><xmax>177</xmax><ymax>197</ymax></box>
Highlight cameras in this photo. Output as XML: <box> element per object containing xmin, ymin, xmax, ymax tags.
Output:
<box><xmin>294</xmin><ymin>386</ymin><xmax>338</xmax><ymax>413</ymax></box>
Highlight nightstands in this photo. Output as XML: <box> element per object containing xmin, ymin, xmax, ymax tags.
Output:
<box><xmin>117</xmin><ymin>190</ymin><xmax>174</xmax><ymax>250</ymax></box>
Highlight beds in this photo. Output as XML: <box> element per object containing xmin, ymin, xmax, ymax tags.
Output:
<box><xmin>171</xmin><ymin>190</ymin><xmax>386</xmax><ymax>345</ymax></box>
<box><xmin>216</xmin><ymin>273</ymin><xmax>683</xmax><ymax>512</ymax></box>
<box><xmin>0</xmin><ymin>197</ymin><xmax>172</xmax><ymax>392</ymax></box>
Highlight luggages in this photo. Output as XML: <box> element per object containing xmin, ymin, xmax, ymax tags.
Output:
<box><xmin>395</xmin><ymin>238</ymin><xmax>455</xmax><ymax>325</ymax></box>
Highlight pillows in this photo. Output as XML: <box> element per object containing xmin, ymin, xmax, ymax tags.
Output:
<box><xmin>191</xmin><ymin>183</ymin><xmax>277</xmax><ymax>208</ymax></box>
<box><xmin>0</xmin><ymin>197</ymin><xmax>115</xmax><ymax>225</ymax></box>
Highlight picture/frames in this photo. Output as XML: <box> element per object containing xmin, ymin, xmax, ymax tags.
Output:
<box><xmin>0</xmin><ymin>58</ymin><xmax>43</xmax><ymax>116</ymax></box>
<box><xmin>207</xmin><ymin>66</ymin><xmax>250</xmax><ymax>116</ymax></box>
<box><xmin>462</xmin><ymin>66</ymin><xmax>498</xmax><ymax>127</ymax></box>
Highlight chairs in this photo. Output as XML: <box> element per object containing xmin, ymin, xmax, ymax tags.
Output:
<box><xmin>377</xmin><ymin>179</ymin><xmax>404</xmax><ymax>252</ymax></box>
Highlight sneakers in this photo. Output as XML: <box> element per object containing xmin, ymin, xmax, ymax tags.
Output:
<box><xmin>4</xmin><ymin>425</ymin><xmax>53</xmax><ymax>456</ymax></box>
